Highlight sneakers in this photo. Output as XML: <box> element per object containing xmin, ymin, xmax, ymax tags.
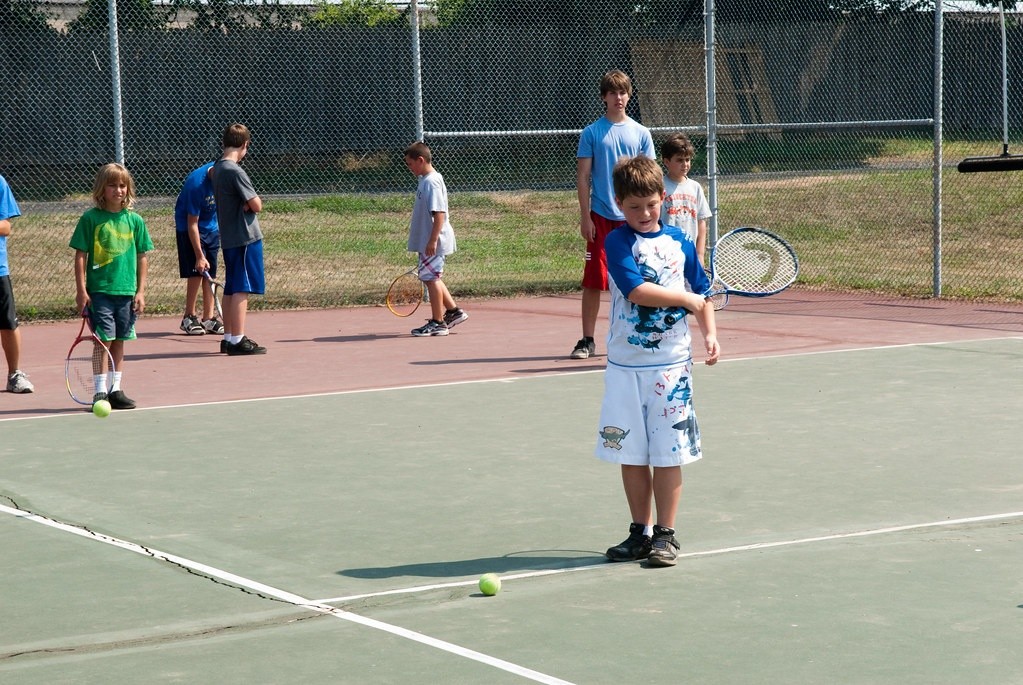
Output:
<box><xmin>5</xmin><ymin>369</ymin><xmax>35</xmax><ymax>394</ymax></box>
<box><xmin>603</xmin><ymin>523</ymin><xmax>653</xmax><ymax>562</ymax></box>
<box><xmin>226</xmin><ymin>336</ymin><xmax>268</xmax><ymax>355</ymax></box>
<box><xmin>178</xmin><ymin>313</ymin><xmax>207</xmax><ymax>336</ymax></box>
<box><xmin>201</xmin><ymin>317</ymin><xmax>226</xmax><ymax>335</ymax></box>
<box><xmin>439</xmin><ymin>306</ymin><xmax>471</xmax><ymax>329</ymax></box>
<box><xmin>410</xmin><ymin>318</ymin><xmax>450</xmax><ymax>337</ymax></box>
<box><xmin>644</xmin><ymin>524</ymin><xmax>682</xmax><ymax>568</ymax></box>
<box><xmin>220</xmin><ymin>340</ymin><xmax>230</xmax><ymax>353</ymax></box>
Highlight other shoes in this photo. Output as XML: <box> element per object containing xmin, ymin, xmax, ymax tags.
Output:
<box><xmin>109</xmin><ymin>390</ymin><xmax>136</xmax><ymax>409</ymax></box>
<box><xmin>90</xmin><ymin>392</ymin><xmax>110</xmax><ymax>412</ymax></box>
<box><xmin>569</xmin><ymin>337</ymin><xmax>597</xmax><ymax>359</ymax></box>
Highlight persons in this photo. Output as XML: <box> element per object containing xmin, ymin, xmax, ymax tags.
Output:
<box><xmin>0</xmin><ymin>175</ymin><xmax>35</xmax><ymax>393</ymax></box>
<box><xmin>404</xmin><ymin>141</ymin><xmax>468</xmax><ymax>337</ymax></box>
<box><xmin>68</xmin><ymin>163</ymin><xmax>154</xmax><ymax>410</ymax></box>
<box><xmin>603</xmin><ymin>151</ymin><xmax>720</xmax><ymax>567</ymax></box>
<box><xmin>211</xmin><ymin>122</ymin><xmax>269</xmax><ymax>356</ymax></box>
<box><xmin>570</xmin><ymin>67</ymin><xmax>657</xmax><ymax>359</ymax></box>
<box><xmin>662</xmin><ymin>132</ymin><xmax>713</xmax><ymax>294</ymax></box>
<box><xmin>165</xmin><ymin>162</ymin><xmax>224</xmax><ymax>335</ymax></box>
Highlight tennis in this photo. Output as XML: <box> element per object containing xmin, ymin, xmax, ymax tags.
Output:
<box><xmin>479</xmin><ymin>572</ymin><xmax>501</xmax><ymax>595</ymax></box>
<box><xmin>93</xmin><ymin>400</ymin><xmax>111</xmax><ymax>418</ymax></box>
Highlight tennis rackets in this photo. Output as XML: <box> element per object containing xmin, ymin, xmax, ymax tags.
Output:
<box><xmin>704</xmin><ymin>268</ymin><xmax>729</xmax><ymax>311</ymax></box>
<box><xmin>203</xmin><ymin>269</ymin><xmax>225</xmax><ymax>321</ymax></box>
<box><xmin>386</xmin><ymin>264</ymin><xmax>424</xmax><ymax>317</ymax></box>
<box><xmin>65</xmin><ymin>303</ymin><xmax>115</xmax><ymax>405</ymax></box>
<box><xmin>664</xmin><ymin>227</ymin><xmax>799</xmax><ymax>326</ymax></box>
